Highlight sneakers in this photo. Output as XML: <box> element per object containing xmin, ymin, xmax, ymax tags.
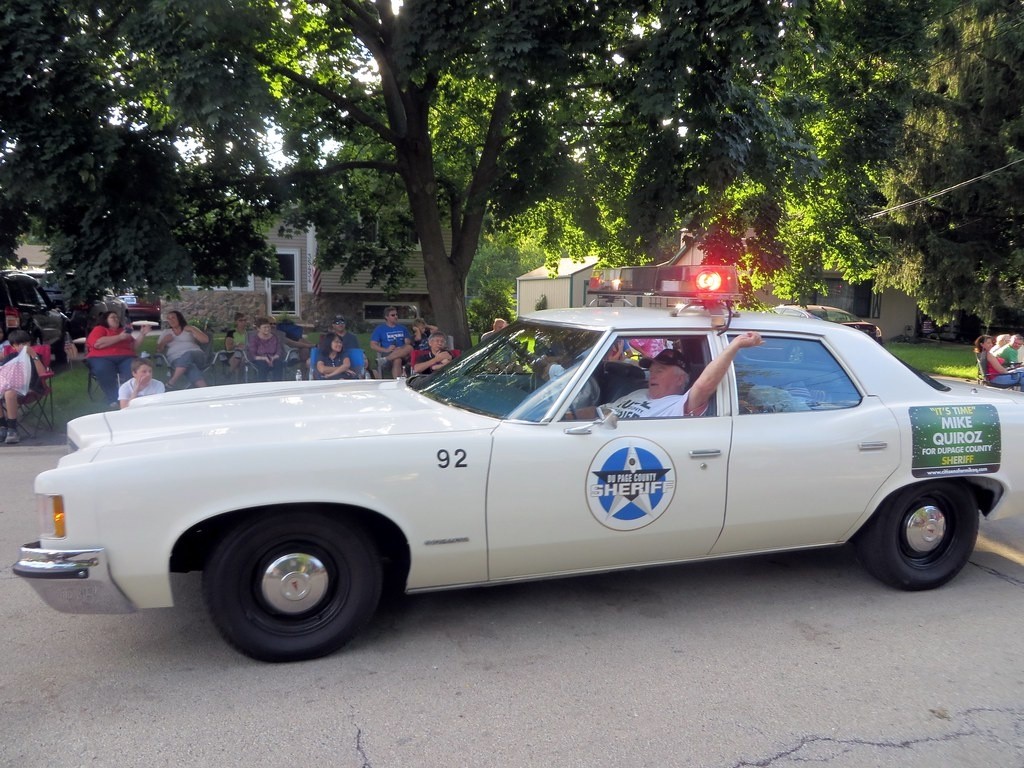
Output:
<box><xmin>374</xmin><ymin>358</ymin><xmax>384</xmax><ymax>367</ymax></box>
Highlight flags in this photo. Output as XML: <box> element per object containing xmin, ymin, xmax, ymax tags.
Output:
<box><xmin>312</xmin><ymin>252</ymin><xmax>322</xmax><ymax>296</ymax></box>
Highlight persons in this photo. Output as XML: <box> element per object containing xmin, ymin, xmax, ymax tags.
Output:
<box><xmin>224</xmin><ymin>312</ymin><xmax>317</xmax><ymax>382</ymax></box>
<box><xmin>973</xmin><ymin>333</ymin><xmax>1024</xmax><ymax>392</ymax></box>
<box><xmin>565</xmin><ymin>331</ymin><xmax>767</xmax><ymax>421</ymax></box>
<box><xmin>537</xmin><ymin>330</ymin><xmax>675</xmax><ymax>413</ymax></box>
<box><xmin>0</xmin><ymin>328</ymin><xmax>46</xmax><ymax>444</ymax></box>
<box><xmin>411</xmin><ymin>318</ymin><xmax>438</xmax><ymax>351</ymax></box>
<box><xmin>117</xmin><ymin>358</ymin><xmax>165</xmax><ymax>409</ymax></box>
<box><xmin>317</xmin><ymin>332</ymin><xmax>359</xmax><ymax>379</ymax></box>
<box><xmin>157</xmin><ymin>311</ymin><xmax>210</xmax><ymax>392</ymax></box>
<box><xmin>85</xmin><ymin>311</ymin><xmax>152</xmax><ymax>407</ymax></box>
<box><xmin>413</xmin><ymin>331</ymin><xmax>452</xmax><ymax>375</ymax></box>
<box><xmin>319</xmin><ymin>314</ymin><xmax>359</xmax><ymax>348</ymax></box>
<box><xmin>369</xmin><ymin>307</ymin><xmax>414</xmax><ymax>379</ymax></box>
<box><xmin>364</xmin><ymin>353</ymin><xmax>379</xmax><ymax>379</ymax></box>
<box><xmin>480</xmin><ymin>319</ymin><xmax>522</xmax><ymax>373</ymax></box>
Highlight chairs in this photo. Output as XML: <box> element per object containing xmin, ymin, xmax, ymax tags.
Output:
<box><xmin>308</xmin><ymin>347</ymin><xmax>366</xmax><ymax>380</ymax></box>
<box><xmin>80</xmin><ymin>359</ymin><xmax>120</xmax><ymax>401</ymax></box>
<box><xmin>594</xmin><ymin>360</ymin><xmax>645</xmax><ymax>404</ymax></box>
<box><xmin>446</xmin><ymin>336</ymin><xmax>454</xmax><ymax>350</ymax></box>
<box><xmin>410</xmin><ymin>349</ymin><xmax>462</xmax><ymax>377</ymax></box>
<box><xmin>685</xmin><ymin>364</ymin><xmax>715</xmax><ymax>415</ymax></box>
<box><xmin>234</xmin><ymin>328</ymin><xmax>300</xmax><ymax>383</ymax></box>
<box><xmin>154</xmin><ymin>331</ymin><xmax>217</xmax><ymax>390</ymax></box>
<box><xmin>515</xmin><ymin>341</ymin><xmax>529</xmax><ymax>366</ymax></box>
<box><xmin>975</xmin><ymin>352</ymin><xmax>1023</xmax><ymax>390</ymax></box>
<box><xmin>276</xmin><ymin>323</ymin><xmax>303</xmax><ymax>340</ymax></box>
<box><xmin>0</xmin><ymin>344</ymin><xmax>55</xmax><ymax>440</ymax></box>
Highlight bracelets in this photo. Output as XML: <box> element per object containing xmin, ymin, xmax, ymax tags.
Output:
<box><xmin>34</xmin><ymin>355</ymin><xmax>40</xmax><ymax>360</ymax></box>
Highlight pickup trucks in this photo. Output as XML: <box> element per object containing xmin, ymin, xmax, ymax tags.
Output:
<box><xmin>0</xmin><ymin>269</ymin><xmax>68</xmax><ymax>365</ymax></box>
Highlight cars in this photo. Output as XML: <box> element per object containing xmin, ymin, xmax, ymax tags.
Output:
<box><xmin>10</xmin><ymin>266</ymin><xmax>1024</xmax><ymax>663</ymax></box>
<box><xmin>20</xmin><ymin>269</ymin><xmax>160</xmax><ymax>351</ymax></box>
<box><xmin>760</xmin><ymin>303</ymin><xmax>883</xmax><ymax>347</ymax></box>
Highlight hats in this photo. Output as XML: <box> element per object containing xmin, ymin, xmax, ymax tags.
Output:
<box><xmin>638</xmin><ymin>349</ymin><xmax>690</xmax><ymax>375</ymax></box>
<box><xmin>332</xmin><ymin>314</ymin><xmax>345</xmax><ymax>324</ymax></box>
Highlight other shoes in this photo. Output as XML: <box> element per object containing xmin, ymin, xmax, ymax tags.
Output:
<box><xmin>165</xmin><ymin>382</ymin><xmax>176</xmax><ymax>390</ymax></box>
<box><xmin>4</xmin><ymin>429</ymin><xmax>21</xmax><ymax>444</ymax></box>
<box><xmin>0</xmin><ymin>426</ymin><xmax>8</xmax><ymax>442</ymax></box>
<box><xmin>110</xmin><ymin>401</ymin><xmax>119</xmax><ymax>408</ymax></box>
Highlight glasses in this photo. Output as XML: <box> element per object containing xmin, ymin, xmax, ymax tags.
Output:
<box><xmin>388</xmin><ymin>313</ymin><xmax>398</xmax><ymax>316</ymax></box>
<box><xmin>107</xmin><ymin>314</ymin><xmax>119</xmax><ymax>318</ymax></box>
<box><xmin>335</xmin><ymin>321</ymin><xmax>345</xmax><ymax>325</ymax></box>
